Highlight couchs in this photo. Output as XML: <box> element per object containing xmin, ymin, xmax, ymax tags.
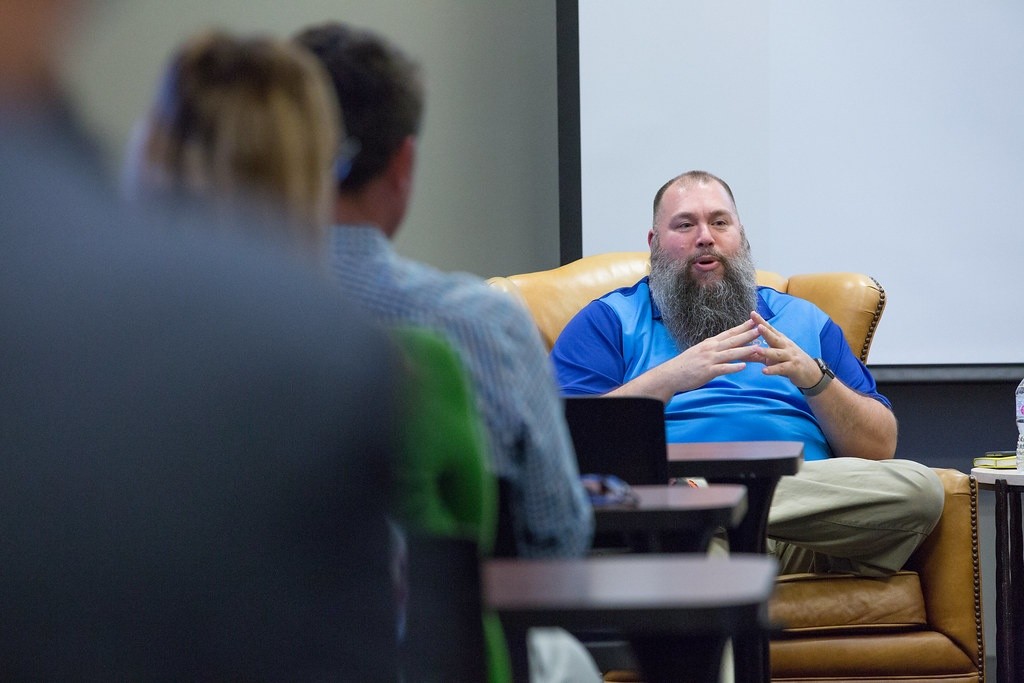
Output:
<box><xmin>487</xmin><ymin>252</ymin><xmax>987</xmax><ymax>683</ymax></box>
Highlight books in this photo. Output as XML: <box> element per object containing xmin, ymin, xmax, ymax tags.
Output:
<box><xmin>973</xmin><ymin>456</ymin><xmax>1016</xmax><ymax>469</ymax></box>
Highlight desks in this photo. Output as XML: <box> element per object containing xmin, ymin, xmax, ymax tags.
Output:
<box><xmin>970</xmin><ymin>466</ymin><xmax>1024</xmax><ymax>683</ymax></box>
<box><xmin>589</xmin><ymin>485</ymin><xmax>748</xmax><ymax>557</ymax></box>
<box><xmin>487</xmin><ymin>556</ymin><xmax>780</xmax><ymax>683</ymax></box>
<box><xmin>666</xmin><ymin>441</ymin><xmax>804</xmax><ymax>557</ymax></box>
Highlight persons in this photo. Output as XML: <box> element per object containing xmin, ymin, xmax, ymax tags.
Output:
<box><xmin>127</xmin><ymin>25</ymin><xmax>508</xmax><ymax>683</ymax></box>
<box><xmin>286</xmin><ymin>18</ymin><xmax>602</xmax><ymax>683</ymax></box>
<box><xmin>552</xmin><ymin>169</ymin><xmax>946</xmax><ymax>583</ymax></box>
<box><xmin>0</xmin><ymin>0</ymin><xmax>413</xmax><ymax>683</ymax></box>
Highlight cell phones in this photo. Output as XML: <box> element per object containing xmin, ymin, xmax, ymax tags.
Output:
<box><xmin>986</xmin><ymin>451</ymin><xmax>1016</xmax><ymax>456</ymax></box>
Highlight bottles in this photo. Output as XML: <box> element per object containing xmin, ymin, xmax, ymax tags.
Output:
<box><xmin>1015</xmin><ymin>379</ymin><xmax>1024</xmax><ymax>471</ymax></box>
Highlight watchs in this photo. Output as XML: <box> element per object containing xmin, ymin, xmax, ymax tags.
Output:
<box><xmin>797</xmin><ymin>357</ymin><xmax>834</xmax><ymax>398</ymax></box>
